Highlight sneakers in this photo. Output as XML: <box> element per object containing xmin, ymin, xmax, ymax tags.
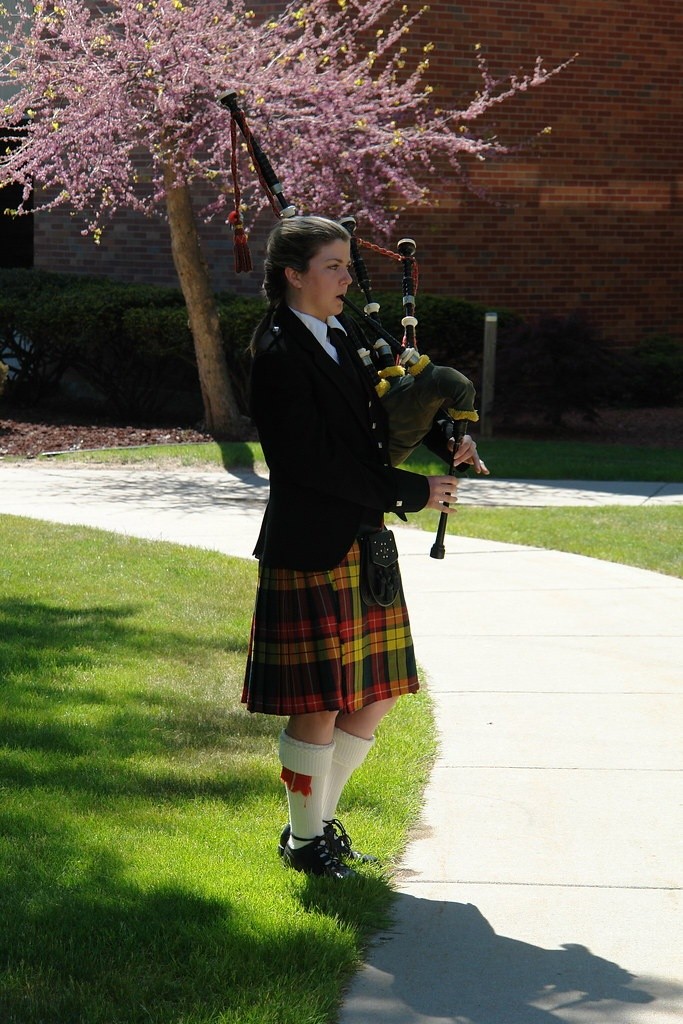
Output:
<box><xmin>283</xmin><ymin>831</ymin><xmax>364</xmax><ymax>884</ymax></box>
<box><xmin>278</xmin><ymin>819</ymin><xmax>378</xmax><ymax>868</ymax></box>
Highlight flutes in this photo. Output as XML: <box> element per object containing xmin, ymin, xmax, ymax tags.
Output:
<box><xmin>212</xmin><ymin>87</ymin><xmax>478</xmax><ymax>560</ymax></box>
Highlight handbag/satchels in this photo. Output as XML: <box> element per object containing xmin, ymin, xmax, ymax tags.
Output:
<box><xmin>361</xmin><ymin>529</ymin><xmax>400</xmax><ymax>608</ymax></box>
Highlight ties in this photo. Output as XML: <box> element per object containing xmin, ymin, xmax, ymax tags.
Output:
<box><xmin>327</xmin><ymin>325</ymin><xmax>362</xmax><ymax>384</ymax></box>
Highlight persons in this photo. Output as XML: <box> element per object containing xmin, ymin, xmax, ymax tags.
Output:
<box><xmin>241</xmin><ymin>216</ymin><xmax>489</xmax><ymax>881</ymax></box>
